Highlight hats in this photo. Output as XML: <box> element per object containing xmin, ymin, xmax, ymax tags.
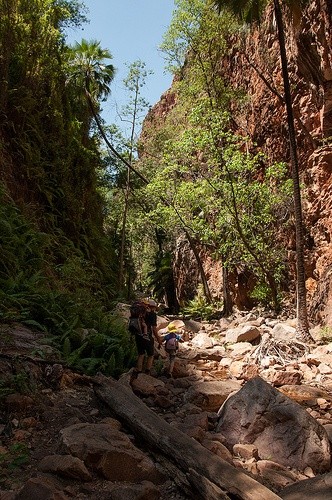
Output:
<box><xmin>166</xmin><ymin>324</ymin><xmax>177</xmax><ymax>334</ymax></box>
<box><xmin>149</xmin><ymin>300</ymin><xmax>157</xmax><ymax>307</ymax></box>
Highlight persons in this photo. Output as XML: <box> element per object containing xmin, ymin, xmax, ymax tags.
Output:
<box><xmin>128</xmin><ymin>299</ymin><xmax>163</xmax><ymax>379</ymax></box>
<box><xmin>157</xmin><ymin>324</ymin><xmax>184</xmax><ymax>378</ymax></box>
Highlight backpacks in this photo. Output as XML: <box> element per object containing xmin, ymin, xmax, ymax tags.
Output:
<box><xmin>166</xmin><ymin>338</ymin><xmax>177</xmax><ymax>349</ymax></box>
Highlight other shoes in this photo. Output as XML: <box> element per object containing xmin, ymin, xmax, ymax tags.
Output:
<box><xmin>137</xmin><ymin>371</ymin><xmax>143</xmax><ymax>374</ymax></box>
<box><xmin>144</xmin><ymin>369</ymin><xmax>153</xmax><ymax>376</ymax></box>
<box><xmin>143</xmin><ymin>333</ymin><xmax>150</xmax><ymax>341</ymax></box>
<box><xmin>168</xmin><ymin>371</ymin><xmax>173</xmax><ymax>377</ymax></box>
